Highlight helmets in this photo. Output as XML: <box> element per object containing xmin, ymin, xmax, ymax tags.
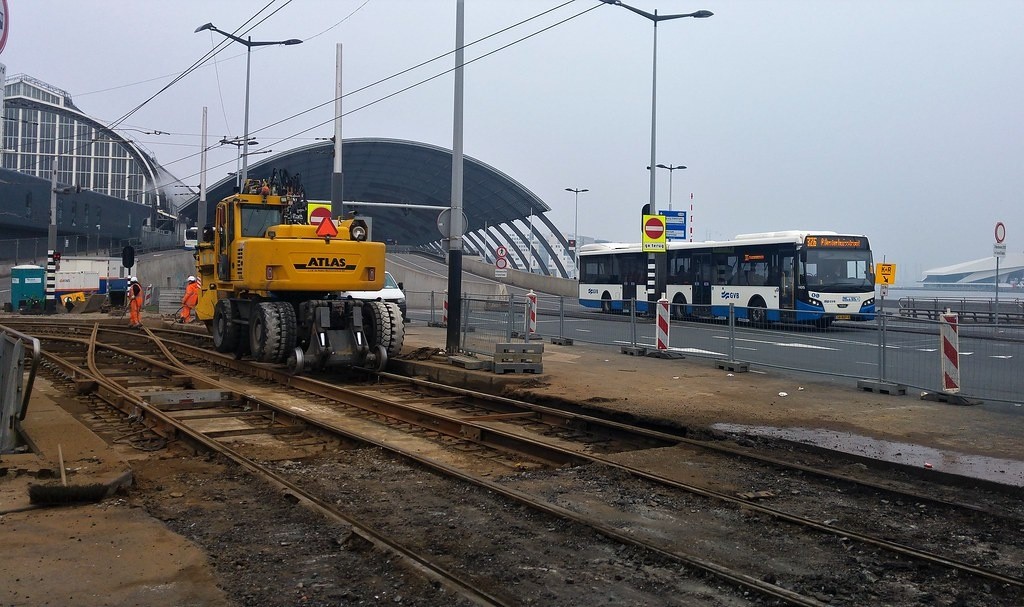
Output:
<box><xmin>188</xmin><ymin>275</ymin><xmax>196</xmax><ymax>281</ymax></box>
<box><xmin>131</xmin><ymin>277</ymin><xmax>137</xmax><ymax>282</ymax></box>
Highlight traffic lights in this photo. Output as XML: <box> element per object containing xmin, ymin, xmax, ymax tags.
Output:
<box><xmin>53</xmin><ymin>253</ymin><xmax>60</xmax><ymax>259</ymax></box>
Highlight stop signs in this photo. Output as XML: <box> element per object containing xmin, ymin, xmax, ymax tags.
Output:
<box><xmin>643</xmin><ymin>215</ymin><xmax>667</xmax><ymax>253</ymax></box>
<box><xmin>308</xmin><ymin>204</ymin><xmax>332</xmax><ymax>225</ymax></box>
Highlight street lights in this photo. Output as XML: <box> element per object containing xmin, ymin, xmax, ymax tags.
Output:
<box><xmin>565</xmin><ymin>188</ymin><xmax>589</xmax><ymax>279</ymax></box>
<box><xmin>655</xmin><ymin>164</ymin><xmax>687</xmax><ymax>242</ymax></box>
<box><xmin>600</xmin><ymin>0</ymin><xmax>714</xmax><ymax>318</ymax></box>
<box><xmin>193</xmin><ymin>23</ymin><xmax>304</xmax><ymax>193</ymax></box>
<box><xmin>220</xmin><ymin>139</ymin><xmax>259</xmax><ymax>194</ymax></box>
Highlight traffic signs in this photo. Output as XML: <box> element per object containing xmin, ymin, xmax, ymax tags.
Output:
<box><xmin>876</xmin><ymin>263</ymin><xmax>895</xmax><ymax>284</ymax></box>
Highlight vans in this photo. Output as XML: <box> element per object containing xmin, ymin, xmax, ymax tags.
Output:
<box><xmin>340</xmin><ymin>271</ymin><xmax>405</xmax><ymax>321</ymax></box>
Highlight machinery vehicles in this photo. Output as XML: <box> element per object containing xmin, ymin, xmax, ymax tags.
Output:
<box><xmin>194</xmin><ymin>170</ymin><xmax>404</xmax><ymax>375</ymax></box>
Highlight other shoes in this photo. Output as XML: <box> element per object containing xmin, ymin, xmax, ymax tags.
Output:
<box><xmin>179</xmin><ymin>317</ymin><xmax>185</xmax><ymax>323</ymax></box>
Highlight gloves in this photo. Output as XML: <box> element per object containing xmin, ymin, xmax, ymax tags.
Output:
<box><xmin>131</xmin><ymin>295</ymin><xmax>135</xmax><ymax>299</ymax></box>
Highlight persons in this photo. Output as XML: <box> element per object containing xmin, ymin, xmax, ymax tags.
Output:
<box><xmin>129</xmin><ymin>276</ymin><xmax>143</xmax><ymax>328</ymax></box>
<box><xmin>179</xmin><ymin>276</ymin><xmax>198</xmax><ymax>323</ymax></box>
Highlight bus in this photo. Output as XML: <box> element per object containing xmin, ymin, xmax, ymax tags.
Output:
<box><xmin>577</xmin><ymin>230</ymin><xmax>875</xmax><ymax>330</ymax></box>
<box><xmin>184</xmin><ymin>227</ymin><xmax>222</xmax><ymax>251</ymax></box>
